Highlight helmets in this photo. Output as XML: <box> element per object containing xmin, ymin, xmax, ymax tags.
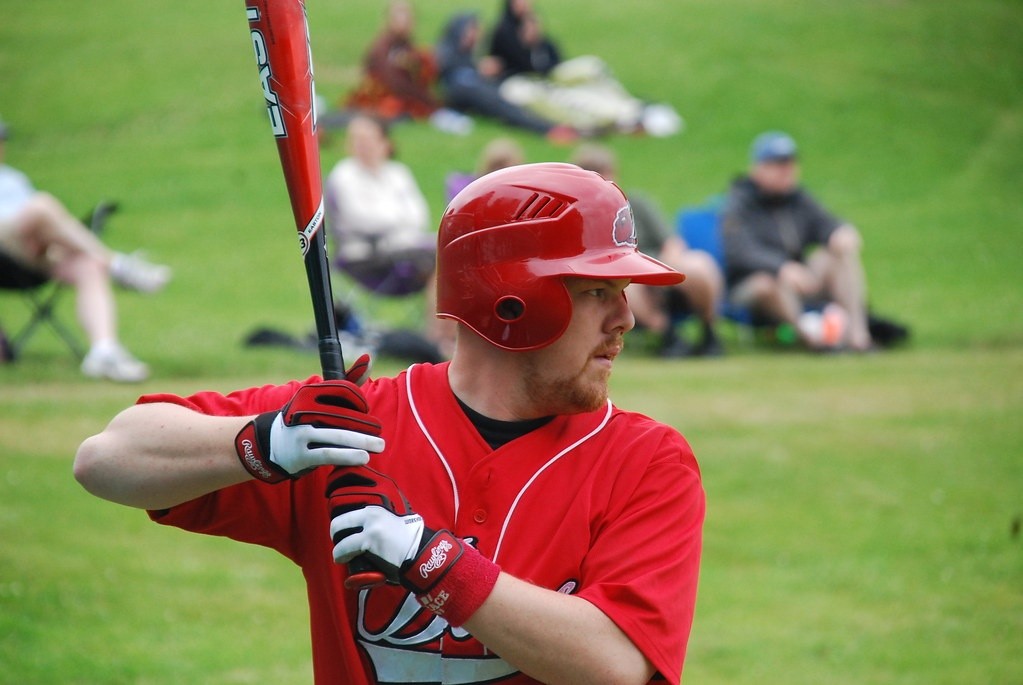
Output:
<box><xmin>436</xmin><ymin>162</ymin><xmax>686</xmax><ymax>352</ymax></box>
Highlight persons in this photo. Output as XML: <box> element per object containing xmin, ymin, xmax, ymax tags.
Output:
<box><xmin>568</xmin><ymin>145</ymin><xmax>728</xmax><ymax>356</ymax></box>
<box><xmin>676</xmin><ymin>131</ymin><xmax>908</xmax><ymax>350</ymax></box>
<box><xmin>0</xmin><ymin>164</ymin><xmax>171</xmax><ymax>382</ymax></box>
<box><xmin>315</xmin><ymin>0</ymin><xmax>681</xmax><ymax>139</ymax></box>
<box><xmin>445</xmin><ymin>140</ymin><xmax>520</xmax><ymax>201</ymax></box>
<box><xmin>322</xmin><ymin>117</ymin><xmax>440</xmax><ymax>299</ymax></box>
<box><xmin>74</xmin><ymin>162</ymin><xmax>704</xmax><ymax>685</ymax></box>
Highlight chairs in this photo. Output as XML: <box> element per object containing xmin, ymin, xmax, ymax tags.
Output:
<box><xmin>0</xmin><ymin>200</ymin><xmax>119</xmax><ymax>363</ymax></box>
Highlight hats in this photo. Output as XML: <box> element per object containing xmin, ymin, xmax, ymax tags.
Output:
<box><xmin>755</xmin><ymin>132</ymin><xmax>797</xmax><ymax>160</ymax></box>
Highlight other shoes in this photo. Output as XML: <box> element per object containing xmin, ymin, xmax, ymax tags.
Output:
<box><xmin>107</xmin><ymin>255</ymin><xmax>171</xmax><ymax>292</ymax></box>
<box><xmin>654</xmin><ymin>334</ymin><xmax>689</xmax><ymax>357</ymax></box>
<box><xmin>697</xmin><ymin>332</ymin><xmax>721</xmax><ymax>355</ymax></box>
<box><xmin>81</xmin><ymin>344</ymin><xmax>145</xmax><ymax>383</ymax></box>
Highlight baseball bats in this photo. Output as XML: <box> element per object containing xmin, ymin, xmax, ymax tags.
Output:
<box><xmin>241</xmin><ymin>2</ymin><xmax>388</xmax><ymax>592</ymax></box>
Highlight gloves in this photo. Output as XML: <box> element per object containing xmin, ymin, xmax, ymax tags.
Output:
<box><xmin>325</xmin><ymin>465</ymin><xmax>463</xmax><ymax>597</ymax></box>
<box><xmin>234</xmin><ymin>354</ymin><xmax>385</xmax><ymax>485</ymax></box>
